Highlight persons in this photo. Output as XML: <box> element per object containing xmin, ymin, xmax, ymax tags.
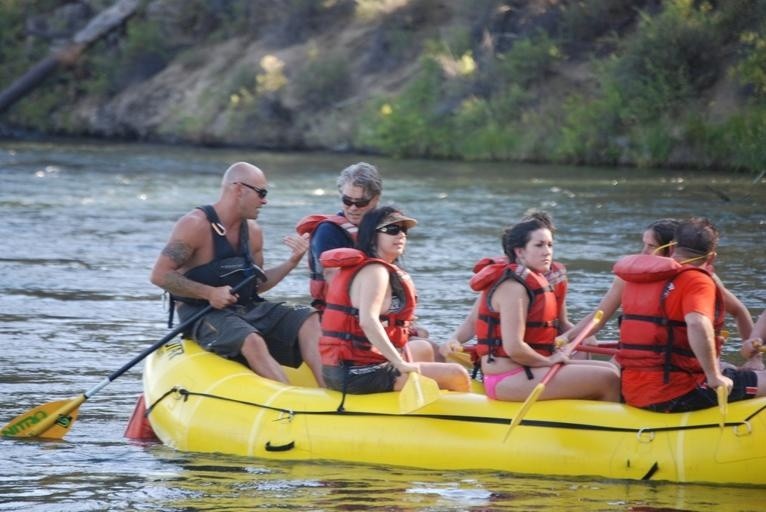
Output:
<box><xmin>468</xmin><ymin>219</ymin><xmax>622</xmax><ymax>402</ymax></box>
<box><xmin>613</xmin><ymin>215</ymin><xmax>766</xmax><ymax>413</ymax></box>
<box><xmin>555</xmin><ymin>219</ymin><xmax>765</xmax><ymax>372</ymax></box>
<box><xmin>318</xmin><ymin>207</ymin><xmax>472</xmax><ymax>392</ymax></box>
<box><xmin>295</xmin><ymin>161</ymin><xmax>447</xmax><ymax>363</ymax></box>
<box><xmin>438</xmin><ymin>207</ymin><xmax>593</xmax><ymax>360</ymax></box>
<box><xmin>151</xmin><ymin>160</ymin><xmax>329</xmax><ymax>389</ymax></box>
<box><xmin>740</xmin><ymin>308</ymin><xmax>766</xmax><ymax>361</ymax></box>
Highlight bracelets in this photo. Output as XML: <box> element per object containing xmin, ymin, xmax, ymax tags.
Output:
<box><xmin>559</xmin><ymin>334</ymin><xmax>570</xmax><ymax>342</ymax></box>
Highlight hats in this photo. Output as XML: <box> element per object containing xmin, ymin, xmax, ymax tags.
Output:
<box><xmin>375</xmin><ymin>212</ymin><xmax>418</xmax><ymax>229</ymax></box>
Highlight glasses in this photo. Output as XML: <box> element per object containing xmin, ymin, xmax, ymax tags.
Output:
<box><xmin>230</xmin><ymin>181</ymin><xmax>267</xmax><ymax>198</ymax></box>
<box><xmin>342</xmin><ymin>194</ymin><xmax>375</xmax><ymax>207</ymax></box>
<box><xmin>376</xmin><ymin>224</ymin><xmax>407</xmax><ymax>236</ymax></box>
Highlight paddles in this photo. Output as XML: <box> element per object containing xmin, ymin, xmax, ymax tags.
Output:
<box><xmin>395</xmin><ymin>342</ymin><xmax>440</xmax><ymax>414</ymax></box>
<box><xmin>498</xmin><ymin>309</ymin><xmax>605</xmax><ymax>445</ymax></box>
<box><xmin>2</xmin><ymin>264</ymin><xmax>268</xmax><ymax>439</ymax></box>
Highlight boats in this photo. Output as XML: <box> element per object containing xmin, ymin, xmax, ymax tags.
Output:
<box><xmin>139</xmin><ymin>332</ymin><xmax>766</xmax><ymax>488</ymax></box>
